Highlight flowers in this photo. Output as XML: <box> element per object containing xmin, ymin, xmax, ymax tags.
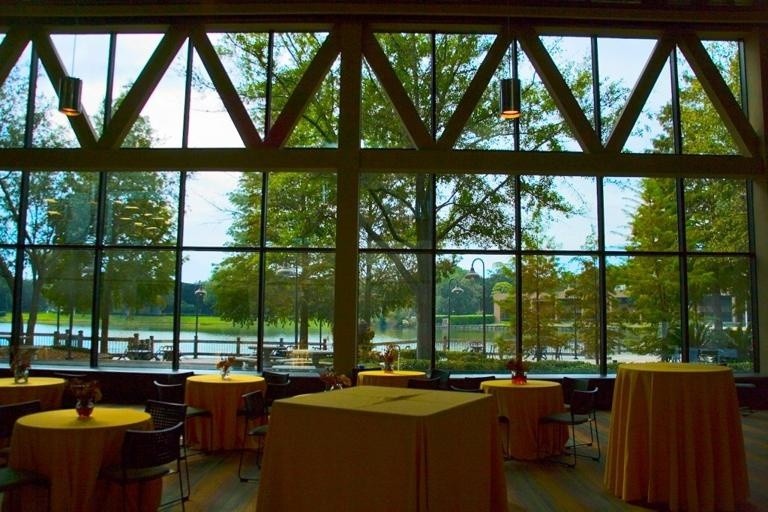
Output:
<box><xmin>211</xmin><ymin>356</ymin><xmax>236</xmax><ymax>377</ymax></box>
<box><xmin>381</xmin><ymin>343</ymin><xmax>400</xmax><ymax>370</ymax></box>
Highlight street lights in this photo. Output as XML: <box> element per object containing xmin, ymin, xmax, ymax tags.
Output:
<box><xmin>465</xmin><ymin>255</ymin><xmax>490</xmax><ymax>355</ymax></box>
<box><xmin>446</xmin><ymin>275</ymin><xmax>463</xmax><ymax>351</ymax></box>
<box><xmin>191</xmin><ymin>283</ymin><xmax>207</xmax><ymax>360</ymax></box>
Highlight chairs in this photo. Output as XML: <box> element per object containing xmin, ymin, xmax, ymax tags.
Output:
<box><xmin>540</xmin><ymin>387</ymin><xmax>603</xmax><ymax>467</ymax></box>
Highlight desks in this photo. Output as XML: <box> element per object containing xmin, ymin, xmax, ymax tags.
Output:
<box><xmin>616</xmin><ymin>362</ymin><xmax>734</xmax><ymax>506</ymax></box>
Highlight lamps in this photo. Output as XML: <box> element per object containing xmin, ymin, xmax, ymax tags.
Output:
<box><xmin>57</xmin><ymin>1</ymin><xmax>83</xmax><ymax>118</ymax></box>
<box><xmin>500</xmin><ymin>1</ymin><xmax>522</xmax><ymax>120</ymax></box>
